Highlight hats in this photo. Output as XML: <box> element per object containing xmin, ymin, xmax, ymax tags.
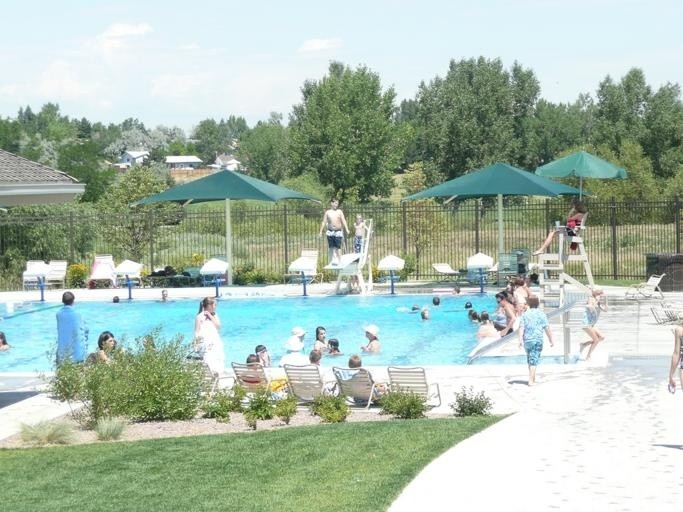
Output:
<box><xmin>284</xmin><ymin>336</ymin><xmax>304</xmax><ymax>351</ymax></box>
<box><xmin>292</xmin><ymin>326</ymin><xmax>307</xmax><ymax>338</ymax></box>
<box><xmin>362</xmin><ymin>325</ymin><xmax>379</xmax><ymax>339</ymax></box>
<box><xmin>329</xmin><ymin>339</ymin><xmax>339</xmax><ymax>347</ymax></box>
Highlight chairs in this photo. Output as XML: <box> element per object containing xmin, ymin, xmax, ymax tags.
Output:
<box><xmin>625</xmin><ymin>273</ymin><xmax>668</xmax><ymax>302</ymax></box>
<box><xmin>177</xmin><ymin>361</ymin><xmax>441</xmax><ymax>410</ymax></box>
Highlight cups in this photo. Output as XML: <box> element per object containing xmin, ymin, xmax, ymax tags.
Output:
<box><xmin>555</xmin><ymin>221</ymin><xmax>561</xmax><ymax>232</ymax></box>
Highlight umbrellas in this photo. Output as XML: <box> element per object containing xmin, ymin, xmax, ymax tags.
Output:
<box><xmin>129</xmin><ymin>168</ymin><xmax>322</xmax><ymax>284</ymax></box>
<box><xmin>535</xmin><ymin>150</ymin><xmax>628</xmax><ymax>200</ymax></box>
<box><xmin>402</xmin><ymin>162</ymin><xmax>590</xmax><ymax>254</ymax></box>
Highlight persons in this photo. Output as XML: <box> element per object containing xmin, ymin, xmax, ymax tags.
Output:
<box><xmin>412</xmin><ymin>305</ymin><xmax>420</xmax><ymax>311</ymax></box>
<box><xmin>580</xmin><ymin>284</ymin><xmax>609</xmax><ymax>362</ymax></box>
<box><xmin>310</xmin><ymin>350</ymin><xmax>338</xmax><ymax>392</ymax></box>
<box><xmin>518</xmin><ymin>252</ymin><xmax>527</xmax><ymax>274</ymax></box>
<box><xmin>58</xmin><ymin>292</ymin><xmax>89</xmax><ymax>400</ymax></box>
<box><xmin>327</xmin><ymin>339</ymin><xmax>343</xmax><ymax>355</ymax></box>
<box><xmin>342</xmin><ymin>356</ymin><xmax>388</xmax><ymax>394</ymax></box>
<box><xmin>668</xmin><ymin>325</ymin><xmax>682</xmax><ymax>394</ymax></box>
<box><xmin>360</xmin><ymin>324</ymin><xmax>382</xmax><ymax>353</ymax></box>
<box><xmin>519</xmin><ymin>295</ymin><xmax>552</xmax><ymax>386</ymax></box>
<box><xmin>532</xmin><ymin>200</ymin><xmax>588</xmax><ymax>256</ymax></box>
<box><xmin>496</xmin><ymin>277</ymin><xmax>548</xmax><ymax>339</ymax></box>
<box><xmin>0</xmin><ymin>331</ymin><xmax>11</xmax><ymax>353</ymax></box>
<box><xmin>433</xmin><ymin>297</ymin><xmax>440</xmax><ymax>305</ymax></box>
<box><xmin>318</xmin><ymin>197</ymin><xmax>350</xmax><ymax>266</ymax></box>
<box><xmin>95</xmin><ymin>331</ymin><xmax>127</xmax><ymax>364</ymax></box>
<box><xmin>465</xmin><ymin>302</ymin><xmax>472</xmax><ymax>308</ymax></box>
<box><xmin>353</xmin><ymin>215</ymin><xmax>369</xmax><ymax>262</ymax></box>
<box><xmin>422</xmin><ymin>311</ymin><xmax>429</xmax><ymax>320</ymax></box>
<box><xmin>256</xmin><ymin>345</ymin><xmax>272</xmax><ymax>367</ymax></box>
<box><xmin>194</xmin><ymin>297</ymin><xmax>224</xmax><ymax>401</ymax></box>
<box><xmin>315</xmin><ymin>326</ymin><xmax>329</xmax><ymax>352</ymax></box>
<box><xmin>162</xmin><ymin>290</ymin><xmax>168</xmax><ymax>301</ymax></box>
<box><xmin>242</xmin><ymin>354</ymin><xmax>293</xmax><ymax>395</ymax></box>
<box><xmin>468</xmin><ymin>310</ymin><xmax>495</xmax><ymax>337</ymax></box>
<box><xmin>278</xmin><ymin>327</ymin><xmax>310</xmax><ymax>366</ymax></box>
<box><xmin>112</xmin><ymin>296</ymin><xmax>119</xmax><ymax>303</ymax></box>
